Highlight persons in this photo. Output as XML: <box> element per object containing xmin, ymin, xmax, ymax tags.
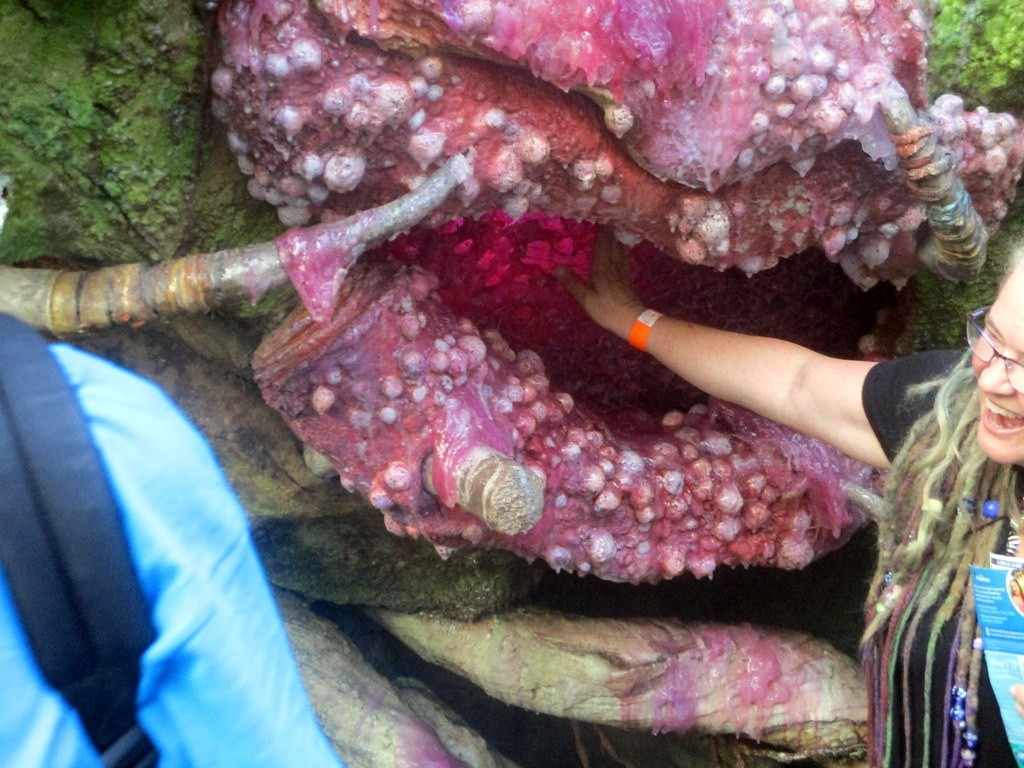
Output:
<box><xmin>0</xmin><ymin>177</ymin><xmax>349</xmax><ymax>768</ymax></box>
<box><xmin>552</xmin><ymin>230</ymin><xmax>1024</xmax><ymax>768</ymax></box>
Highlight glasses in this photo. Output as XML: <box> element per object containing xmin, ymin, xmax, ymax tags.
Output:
<box><xmin>966</xmin><ymin>305</ymin><xmax>1024</xmax><ymax>395</ymax></box>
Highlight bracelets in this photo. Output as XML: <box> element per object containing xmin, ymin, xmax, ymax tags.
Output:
<box><xmin>627</xmin><ymin>308</ymin><xmax>664</xmax><ymax>354</ymax></box>
